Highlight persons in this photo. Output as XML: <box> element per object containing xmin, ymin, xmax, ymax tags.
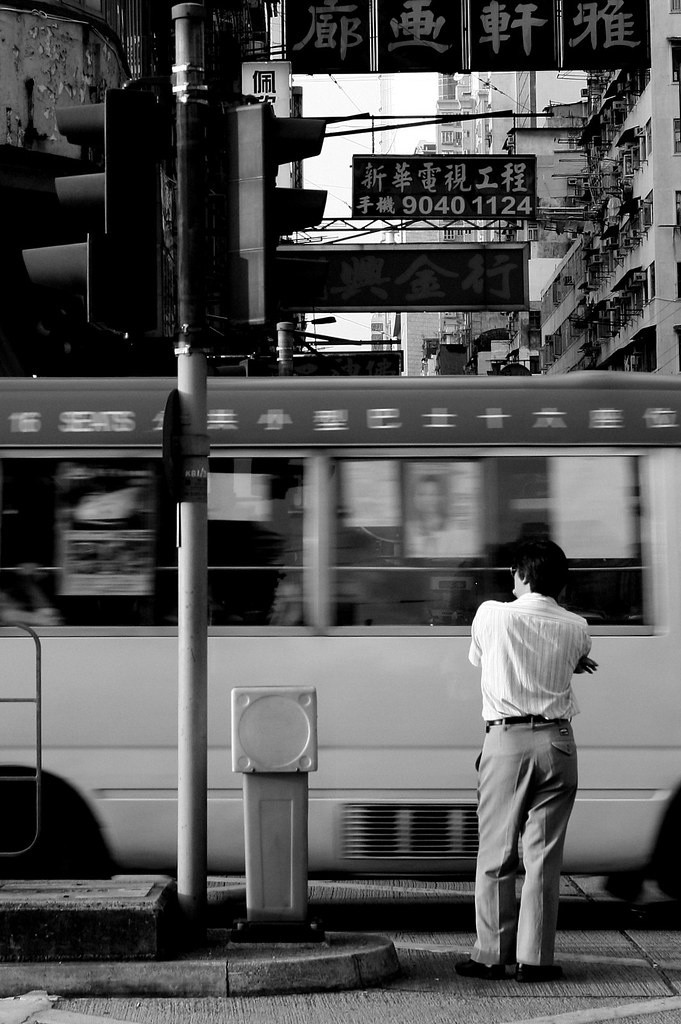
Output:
<box><xmin>406</xmin><ymin>473</ymin><xmax>460</xmax><ymax>561</ymax></box>
<box><xmin>453</xmin><ymin>540</ymin><xmax>601</xmax><ymax>982</ymax></box>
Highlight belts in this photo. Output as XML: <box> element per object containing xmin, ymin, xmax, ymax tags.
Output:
<box><xmin>488</xmin><ymin>716</ymin><xmax>565</xmax><ymax>726</ymax></box>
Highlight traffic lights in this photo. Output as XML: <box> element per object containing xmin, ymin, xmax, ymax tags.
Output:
<box><xmin>21</xmin><ymin>86</ymin><xmax>173</xmax><ymax>338</ymax></box>
<box><xmin>231</xmin><ymin>100</ymin><xmax>327</xmax><ymax>332</ymax></box>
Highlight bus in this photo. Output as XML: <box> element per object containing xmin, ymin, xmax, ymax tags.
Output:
<box><xmin>0</xmin><ymin>371</ymin><xmax>681</xmax><ymax>881</ymax></box>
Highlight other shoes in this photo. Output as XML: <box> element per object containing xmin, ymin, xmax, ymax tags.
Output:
<box><xmin>453</xmin><ymin>959</ymin><xmax>505</xmax><ymax>981</ymax></box>
<box><xmin>514</xmin><ymin>963</ymin><xmax>562</xmax><ymax>982</ymax></box>
